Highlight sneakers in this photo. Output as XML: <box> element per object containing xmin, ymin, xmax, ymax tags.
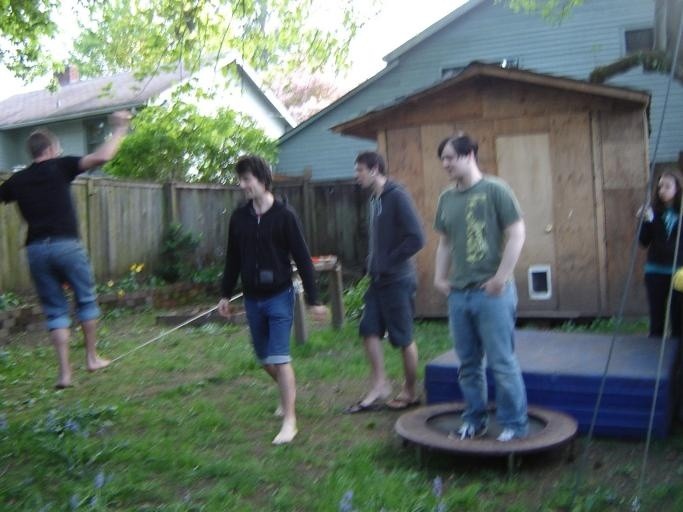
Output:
<box><xmin>448</xmin><ymin>423</ymin><xmax>489</xmax><ymax>442</ymax></box>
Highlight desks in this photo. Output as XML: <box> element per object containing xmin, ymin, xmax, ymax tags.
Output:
<box><xmin>293</xmin><ymin>256</ymin><xmax>345</xmax><ymax>345</ymax></box>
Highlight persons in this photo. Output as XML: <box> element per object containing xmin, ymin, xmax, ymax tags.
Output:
<box><xmin>632</xmin><ymin>166</ymin><xmax>683</xmax><ymax>339</ymax></box>
<box><xmin>0</xmin><ymin>109</ymin><xmax>133</xmax><ymax>388</ymax></box>
<box><xmin>215</xmin><ymin>152</ymin><xmax>330</xmax><ymax>446</ymax></box>
<box><xmin>430</xmin><ymin>131</ymin><xmax>534</xmax><ymax>442</ymax></box>
<box><xmin>341</xmin><ymin>151</ymin><xmax>425</xmax><ymax>415</ymax></box>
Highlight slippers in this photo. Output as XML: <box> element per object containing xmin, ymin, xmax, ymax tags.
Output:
<box><xmin>342</xmin><ymin>399</ymin><xmax>381</xmax><ymax>415</ymax></box>
<box><xmin>385</xmin><ymin>396</ymin><xmax>421</xmax><ymax>411</ymax></box>
